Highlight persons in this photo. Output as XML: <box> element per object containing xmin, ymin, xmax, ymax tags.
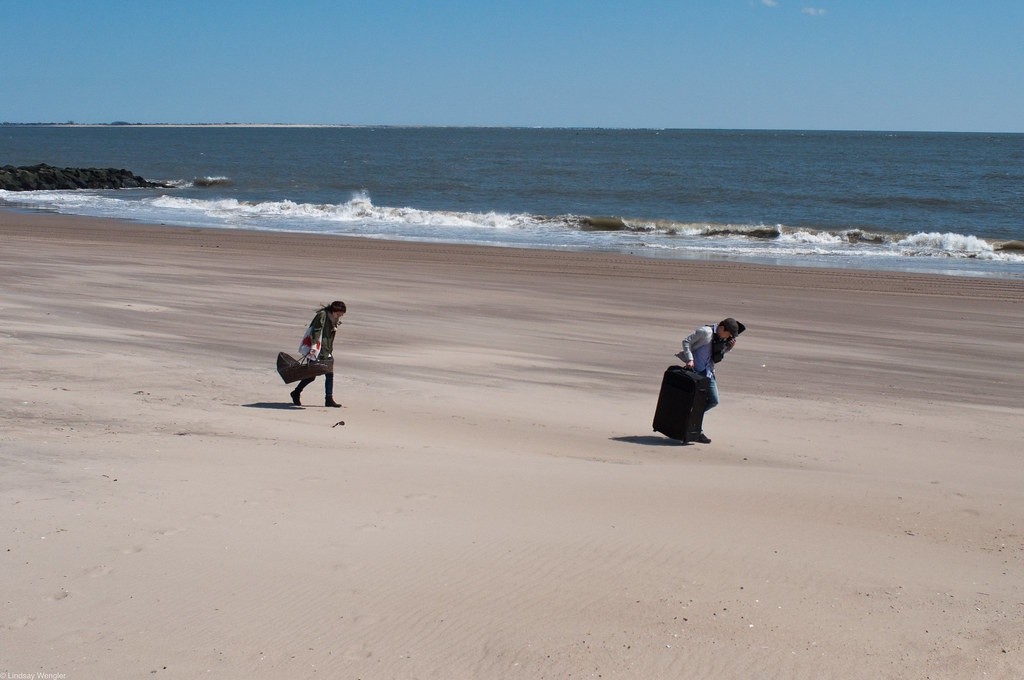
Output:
<box><xmin>674</xmin><ymin>318</ymin><xmax>739</xmax><ymax>444</ymax></box>
<box><xmin>290</xmin><ymin>301</ymin><xmax>346</xmax><ymax>408</ymax></box>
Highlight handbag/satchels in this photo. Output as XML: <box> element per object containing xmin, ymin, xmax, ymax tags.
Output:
<box><xmin>283</xmin><ymin>353</ymin><xmax>324</xmax><ymax>384</ymax></box>
<box><xmin>277</xmin><ymin>352</ymin><xmax>300</xmax><ymax>385</ymax></box>
<box><xmin>318</xmin><ymin>357</ymin><xmax>333</xmax><ymax>373</ymax></box>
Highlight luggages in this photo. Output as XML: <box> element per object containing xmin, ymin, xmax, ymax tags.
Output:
<box><xmin>653</xmin><ymin>365</ymin><xmax>709</xmax><ymax>445</ymax></box>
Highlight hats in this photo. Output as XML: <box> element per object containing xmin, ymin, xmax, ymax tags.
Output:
<box><xmin>723</xmin><ymin>318</ymin><xmax>739</xmax><ymax>337</ymax></box>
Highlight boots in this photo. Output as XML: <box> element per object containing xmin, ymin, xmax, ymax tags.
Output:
<box><xmin>325</xmin><ymin>394</ymin><xmax>341</xmax><ymax>407</ymax></box>
<box><xmin>291</xmin><ymin>387</ymin><xmax>303</xmax><ymax>406</ymax></box>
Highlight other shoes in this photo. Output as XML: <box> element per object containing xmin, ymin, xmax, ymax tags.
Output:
<box><xmin>696</xmin><ymin>433</ymin><xmax>711</xmax><ymax>443</ymax></box>
